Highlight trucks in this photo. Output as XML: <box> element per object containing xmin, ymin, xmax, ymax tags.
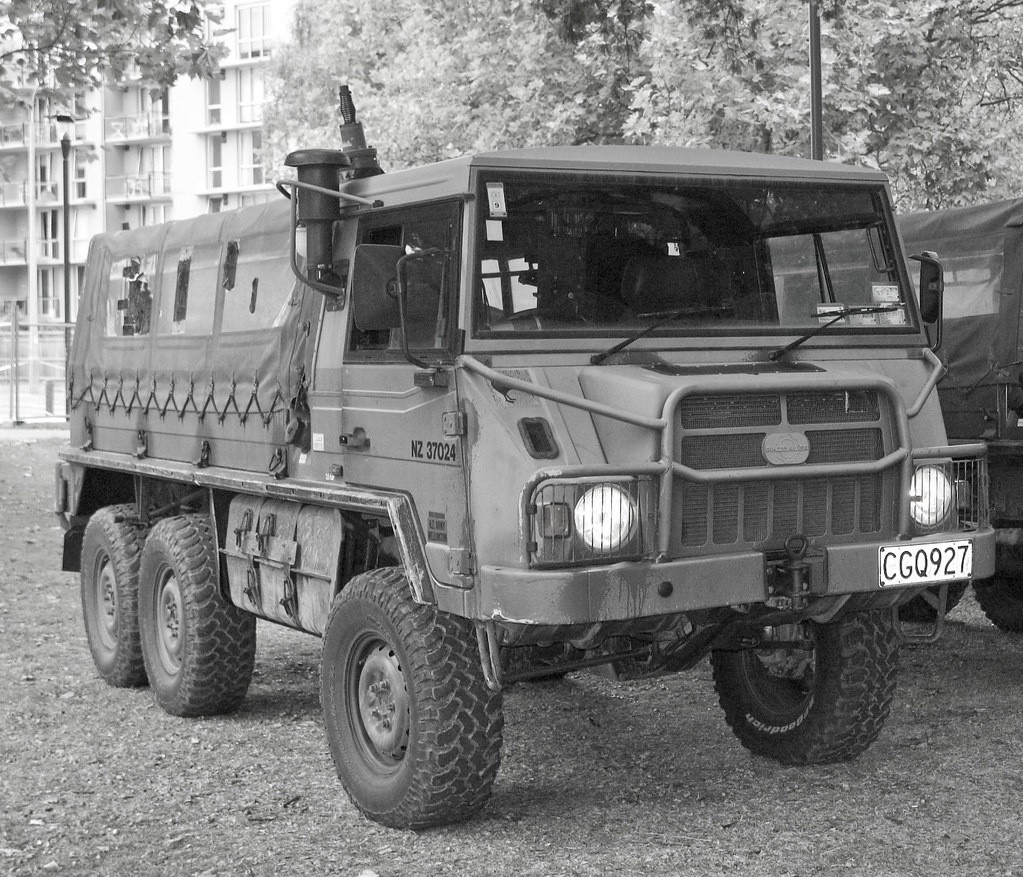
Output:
<box><xmin>767</xmin><ymin>191</ymin><xmax>1023</xmax><ymax>636</ymax></box>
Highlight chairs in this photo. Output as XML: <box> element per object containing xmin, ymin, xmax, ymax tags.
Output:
<box><xmin>125</xmin><ymin>177</ymin><xmax>144</xmax><ymax>195</ymax></box>
<box><xmin>616</xmin><ymin>249</ymin><xmax>707</xmax><ymax>320</ymax></box>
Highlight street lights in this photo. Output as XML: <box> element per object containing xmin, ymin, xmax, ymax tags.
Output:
<box><xmin>55</xmin><ymin>108</ymin><xmax>78</xmax><ymax>424</ymax></box>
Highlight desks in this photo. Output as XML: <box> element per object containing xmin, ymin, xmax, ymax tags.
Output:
<box><xmin>111</xmin><ymin>121</ymin><xmax>126</xmax><ymax>138</ymax></box>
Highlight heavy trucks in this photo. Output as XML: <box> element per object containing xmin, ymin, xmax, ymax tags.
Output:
<box><xmin>48</xmin><ymin>84</ymin><xmax>998</xmax><ymax>831</ymax></box>
